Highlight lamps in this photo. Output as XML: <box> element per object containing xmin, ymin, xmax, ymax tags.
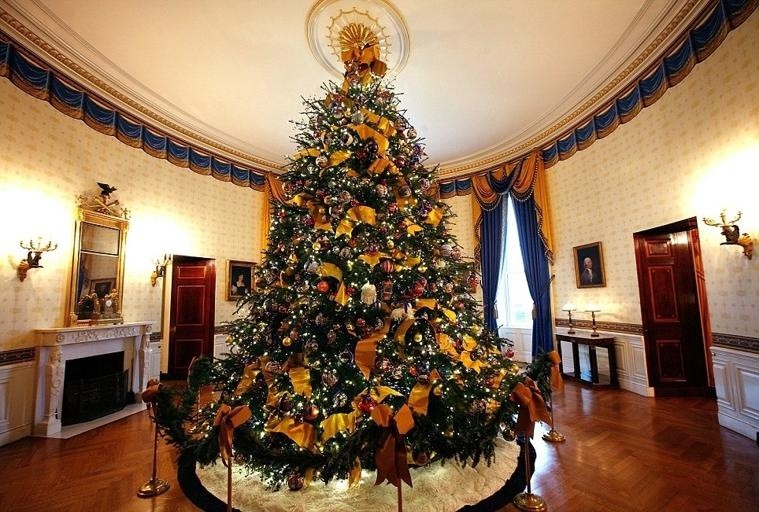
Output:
<box><xmin>561</xmin><ymin>304</ymin><xmax>601</xmax><ymax>336</ymax></box>
<box><xmin>703</xmin><ymin>206</ymin><xmax>756</xmax><ymax>259</ymax></box>
<box><xmin>15</xmin><ymin>234</ymin><xmax>57</xmax><ymax>279</ymax></box>
<box><xmin>150</xmin><ymin>249</ymin><xmax>173</xmax><ymax>284</ymax></box>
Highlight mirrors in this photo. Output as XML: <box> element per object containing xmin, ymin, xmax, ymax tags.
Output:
<box><xmin>67</xmin><ymin>194</ymin><xmax>128</xmax><ymax>325</ymax></box>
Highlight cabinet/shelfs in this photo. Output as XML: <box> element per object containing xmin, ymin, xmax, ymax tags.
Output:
<box><xmin>555</xmin><ymin>334</ymin><xmax>620</xmax><ymax>389</ymax></box>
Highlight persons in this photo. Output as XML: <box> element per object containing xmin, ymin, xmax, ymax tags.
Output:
<box><xmin>580</xmin><ymin>255</ymin><xmax>601</xmax><ymax>285</ymax></box>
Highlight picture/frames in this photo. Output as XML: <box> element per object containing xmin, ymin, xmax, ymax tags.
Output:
<box><xmin>225</xmin><ymin>257</ymin><xmax>257</xmax><ymax>301</ymax></box>
<box><xmin>572</xmin><ymin>241</ymin><xmax>608</xmax><ymax>288</ymax></box>
